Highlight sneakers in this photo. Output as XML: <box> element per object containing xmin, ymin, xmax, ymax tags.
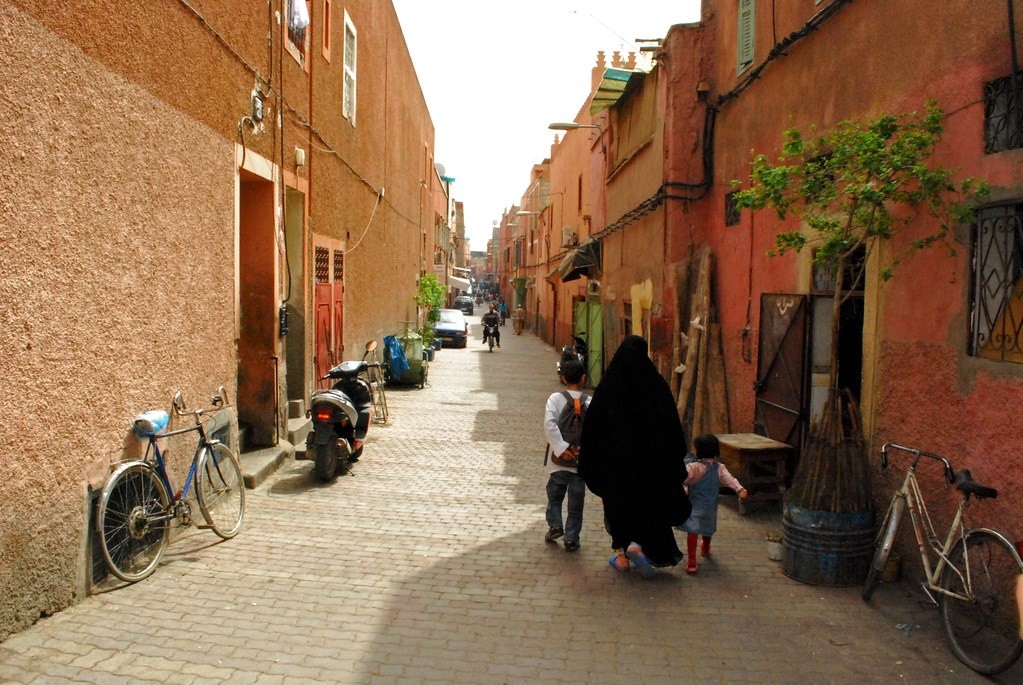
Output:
<box><xmin>545</xmin><ymin>526</ymin><xmax>564</xmax><ymax>541</ymax></box>
<box><xmin>565</xmin><ymin>541</ymin><xmax>579</xmax><ymax>552</ymax></box>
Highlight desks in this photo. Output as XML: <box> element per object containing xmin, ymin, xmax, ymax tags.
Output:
<box><xmin>715</xmin><ymin>433</ymin><xmax>793</xmax><ymax>517</ymax></box>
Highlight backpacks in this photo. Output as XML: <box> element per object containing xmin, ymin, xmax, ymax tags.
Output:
<box><xmin>551</xmin><ymin>391</ymin><xmax>588</xmax><ymax>467</ymax></box>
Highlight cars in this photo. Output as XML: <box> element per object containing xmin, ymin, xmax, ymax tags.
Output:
<box><xmin>425</xmin><ymin>309</ymin><xmax>468</xmax><ymax>347</ymax></box>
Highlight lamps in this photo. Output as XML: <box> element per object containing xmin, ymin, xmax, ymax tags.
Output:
<box><xmin>507</xmin><ymin>223</ymin><xmax>522</xmax><ymax>230</ymax></box>
<box><xmin>548</xmin><ymin>121</ymin><xmax>606</xmax><ymax>154</ymax></box>
<box><xmin>517</xmin><ymin>211</ymin><xmax>546</xmax><ymax>226</ymax></box>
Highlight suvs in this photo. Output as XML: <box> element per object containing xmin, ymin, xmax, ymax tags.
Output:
<box><xmin>452</xmin><ymin>296</ymin><xmax>473</xmax><ymax>315</ymax></box>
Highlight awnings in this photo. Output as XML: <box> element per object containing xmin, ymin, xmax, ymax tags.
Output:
<box><xmin>557</xmin><ymin>239</ymin><xmax>602</xmax><ymax>283</ymax></box>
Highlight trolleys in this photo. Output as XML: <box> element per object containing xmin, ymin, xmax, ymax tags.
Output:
<box><xmin>387</xmin><ymin>351</ymin><xmax>428</xmax><ymax>388</ymax></box>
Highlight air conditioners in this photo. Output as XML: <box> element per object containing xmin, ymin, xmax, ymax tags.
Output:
<box><xmin>561</xmin><ymin>226</ymin><xmax>574</xmax><ymax>248</ymax></box>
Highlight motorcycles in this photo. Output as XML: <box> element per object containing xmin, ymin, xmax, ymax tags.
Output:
<box><xmin>481</xmin><ymin>322</ymin><xmax>500</xmax><ymax>352</ymax></box>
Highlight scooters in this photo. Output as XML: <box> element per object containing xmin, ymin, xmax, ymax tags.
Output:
<box><xmin>306</xmin><ymin>340</ymin><xmax>390</xmax><ymax>480</ymax></box>
<box><xmin>557</xmin><ymin>332</ymin><xmax>586</xmax><ymax>383</ymax></box>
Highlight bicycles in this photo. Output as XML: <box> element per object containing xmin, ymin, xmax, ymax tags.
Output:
<box><xmin>476</xmin><ymin>297</ymin><xmax>480</xmax><ymax>306</ymax></box>
<box><xmin>96</xmin><ymin>386</ymin><xmax>245</xmax><ymax>582</ymax></box>
<box><xmin>861</xmin><ymin>443</ymin><xmax>1023</xmax><ymax>674</ymax></box>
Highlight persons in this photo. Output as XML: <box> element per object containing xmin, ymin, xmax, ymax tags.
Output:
<box><xmin>544</xmin><ymin>360</ymin><xmax>594</xmax><ymax>551</ymax></box>
<box><xmin>472</xmin><ymin>286</ymin><xmax>525</xmax><ymax>349</ymax></box>
<box><xmin>578</xmin><ymin>334</ymin><xmax>692</xmax><ymax>572</ymax></box>
<box><xmin>680</xmin><ymin>432</ymin><xmax>747</xmax><ymax>574</ymax></box>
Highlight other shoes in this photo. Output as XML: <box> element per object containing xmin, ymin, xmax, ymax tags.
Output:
<box><xmin>483</xmin><ymin>339</ymin><xmax>487</xmax><ymax>343</ymax></box>
<box><xmin>497</xmin><ymin>343</ymin><xmax>501</xmax><ymax>348</ymax></box>
<box><xmin>685</xmin><ymin>563</ymin><xmax>699</xmax><ymax>574</ymax></box>
<box><xmin>701</xmin><ymin>542</ymin><xmax>712</xmax><ymax>557</ymax></box>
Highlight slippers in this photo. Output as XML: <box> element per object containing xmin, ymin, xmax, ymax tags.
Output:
<box><xmin>609</xmin><ymin>555</ymin><xmax>631</xmax><ymax>573</ymax></box>
<box><xmin>626</xmin><ymin>546</ymin><xmax>654</xmax><ymax>580</ymax></box>
